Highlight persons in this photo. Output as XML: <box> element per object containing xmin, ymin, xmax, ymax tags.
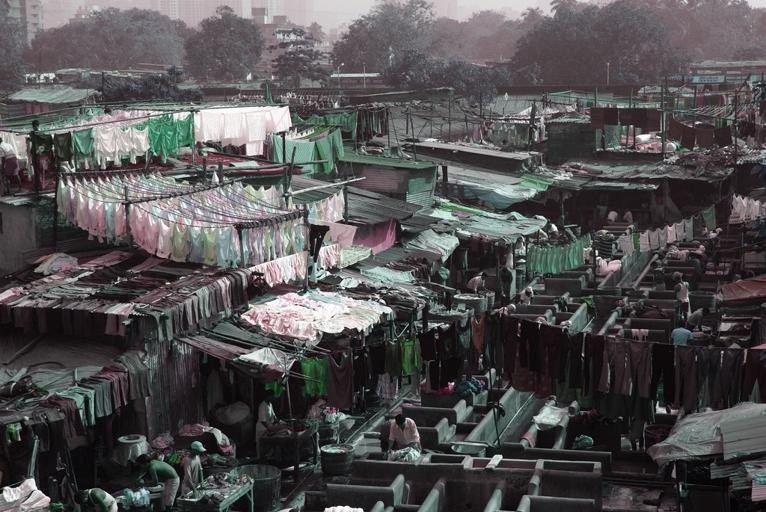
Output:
<box><xmin>466</xmin><ymin>271</ymin><xmax>487</xmax><ymax>295</ymax></box>
<box><xmin>685</xmin><ymin>306</ymin><xmax>708</xmax><ymax>331</ymax></box>
<box><xmin>180</xmin><ymin>440</ymin><xmax>208</xmax><ymax>499</ymax></box>
<box><xmin>0</xmin><ymin>137</ymin><xmax>22</xmax><ymax>197</ymax></box>
<box><xmin>386</xmin><ymin>413</ymin><xmax>421</xmax><ymax>452</ymax></box>
<box><xmin>671</xmin><ymin>320</ymin><xmax>694</xmax><ymax>346</ymax></box>
<box><xmin>73</xmin><ymin>487</ymin><xmax>119</xmax><ymax>512</ymax></box>
<box><xmin>24</xmin><ymin>118</ymin><xmax>48</xmax><ymax>190</ymax></box>
<box><xmin>670</xmin><ymin>271</ymin><xmax>690</xmax><ymax>318</ymax></box>
<box><xmin>500</xmin><ymin>266</ymin><xmax>514</xmax><ymax>302</ymax></box>
<box><xmin>516</xmin><ymin>286</ymin><xmax>535</xmax><ymax>305</ymax></box>
<box><xmin>134</xmin><ymin>453</ymin><xmax>181</xmax><ymax>512</ymax></box>
<box><xmin>253</xmin><ymin>392</ymin><xmax>277</xmax><ymax>461</ymax></box>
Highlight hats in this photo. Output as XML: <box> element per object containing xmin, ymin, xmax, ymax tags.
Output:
<box><xmin>190</xmin><ymin>440</ymin><xmax>207</xmax><ymax>453</ymax></box>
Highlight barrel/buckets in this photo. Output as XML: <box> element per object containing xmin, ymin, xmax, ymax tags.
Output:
<box><xmin>321</xmin><ymin>443</ymin><xmax>355</xmax><ymax>475</ymax></box>
<box><xmin>230</xmin><ymin>463</ymin><xmax>281</xmax><ymax>512</ymax></box>
<box><xmin>645</xmin><ymin>424</ymin><xmax>674</xmax><ymax>448</ymax></box>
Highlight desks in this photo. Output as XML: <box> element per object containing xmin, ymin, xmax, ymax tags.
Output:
<box><xmin>176</xmin><ymin>472</ymin><xmax>255</xmax><ymax>512</ymax></box>
<box><xmin>259</xmin><ymin>418</ymin><xmax>320</xmax><ymax>484</ymax></box>
